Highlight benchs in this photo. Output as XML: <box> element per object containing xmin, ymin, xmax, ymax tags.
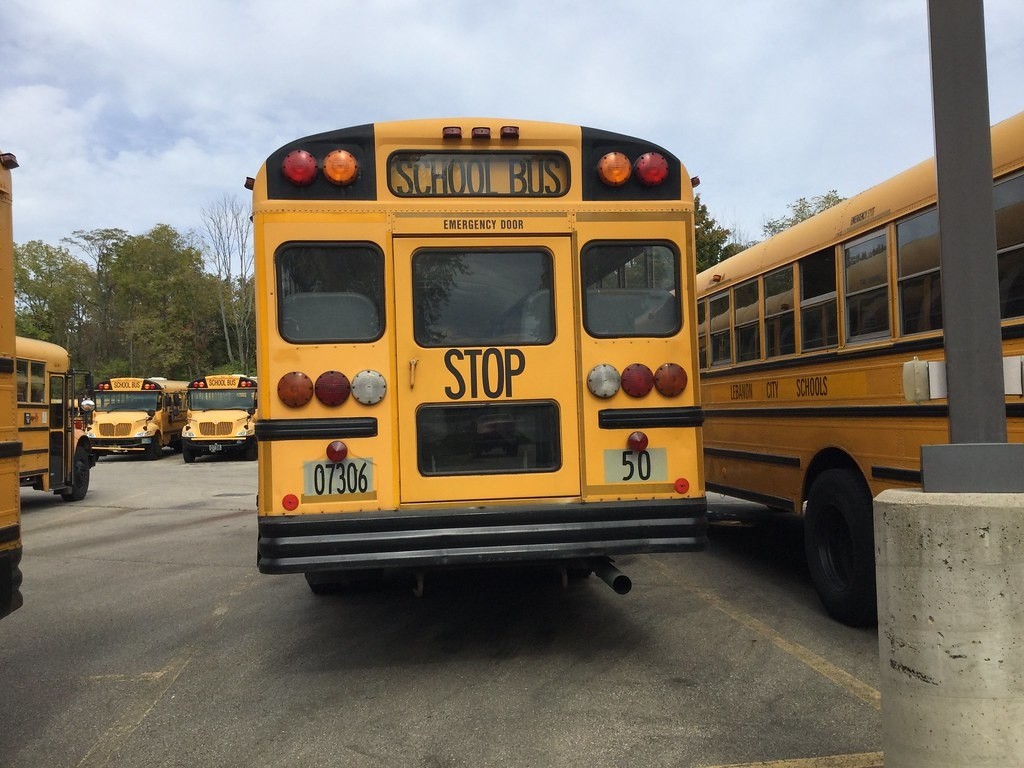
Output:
<box><xmin>520</xmin><ymin>288</ymin><xmax>678</xmax><ymax>338</ymax></box>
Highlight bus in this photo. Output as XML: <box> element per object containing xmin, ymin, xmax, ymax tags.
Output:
<box><xmin>0</xmin><ymin>152</ymin><xmax>24</xmax><ymax>619</ymax></box>
<box><xmin>16</xmin><ymin>336</ymin><xmax>97</xmax><ymax>501</ymax></box>
<box><xmin>697</xmin><ymin>107</ymin><xmax>1023</xmax><ymax>629</ymax></box>
<box><xmin>245</xmin><ymin>117</ymin><xmax>711</xmax><ymax>597</ymax></box>
<box><xmin>73</xmin><ymin>377</ymin><xmax>190</xmax><ymax>463</ymax></box>
<box><xmin>181</xmin><ymin>373</ymin><xmax>259</xmax><ymax>464</ymax></box>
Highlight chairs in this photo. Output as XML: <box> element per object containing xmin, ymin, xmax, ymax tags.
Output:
<box><xmin>281</xmin><ymin>292</ymin><xmax>377</xmax><ymax>342</ymax></box>
